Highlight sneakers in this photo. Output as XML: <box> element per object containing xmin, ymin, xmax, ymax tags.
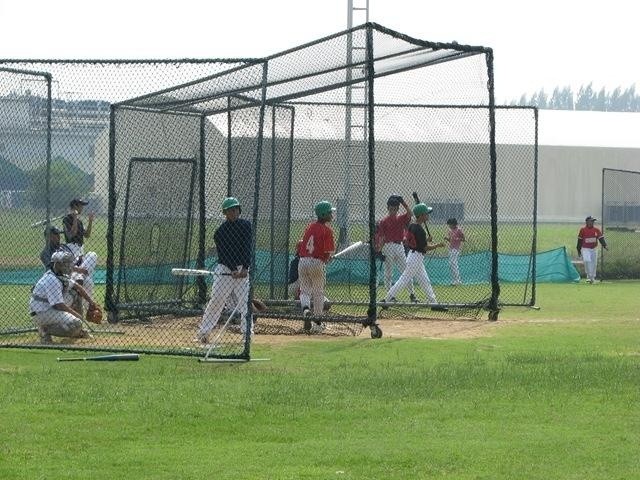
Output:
<box><xmin>39</xmin><ymin>326</ymin><xmax>52</xmax><ymax>344</ymax></box>
<box><xmin>380</xmin><ymin>298</ymin><xmax>401</xmax><ymax>309</ymax></box>
<box><xmin>314</xmin><ymin>322</ymin><xmax>326</xmax><ymax>331</ymax></box>
<box><xmin>411</xmin><ymin>294</ymin><xmax>421</xmax><ymax>303</ymax></box>
<box><xmin>431</xmin><ymin>305</ymin><xmax>449</xmax><ymax>311</ymax></box>
<box><xmin>304</xmin><ymin>310</ymin><xmax>312</xmax><ymax>330</ymax></box>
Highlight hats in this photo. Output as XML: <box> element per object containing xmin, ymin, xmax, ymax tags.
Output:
<box><xmin>44</xmin><ymin>225</ymin><xmax>65</xmax><ymax>235</ymax></box>
<box><xmin>586</xmin><ymin>216</ymin><xmax>596</xmax><ymax>221</ymax></box>
<box><xmin>71</xmin><ymin>197</ymin><xmax>90</xmax><ymax>206</ymax></box>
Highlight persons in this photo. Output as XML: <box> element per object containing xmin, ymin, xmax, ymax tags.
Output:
<box><xmin>576</xmin><ymin>215</ymin><xmax>608</xmax><ymax>283</ymax></box>
<box><xmin>288</xmin><ymin>240</ymin><xmax>332</xmax><ymax>311</ymax></box>
<box><xmin>444</xmin><ymin>217</ymin><xmax>466</xmax><ymax>285</ymax></box>
<box><xmin>376</xmin><ymin>203</ymin><xmax>449</xmax><ymax>311</ymax></box>
<box><xmin>28</xmin><ymin>197</ymin><xmax>103</xmax><ymax>345</ymax></box>
<box><xmin>298</xmin><ymin>200</ymin><xmax>337</xmax><ymax>330</ymax></box>
<box><xmin>193</xmin><ymin>197</ymin><xmax>255</xmax><ymax>344</ymax></box>
<box><xmin>375</xmin><ymin>195</ymin><xmax>421</xmax><ymax>302</ymax></box>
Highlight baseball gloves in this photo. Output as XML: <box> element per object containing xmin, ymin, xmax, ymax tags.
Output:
<box><xmin>86</xmin><ymin>304</ymin><xmax>102</xmax><ymax>325</ymax></box>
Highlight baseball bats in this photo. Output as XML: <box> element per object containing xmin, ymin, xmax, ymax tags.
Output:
<box><xmin>31</xmin><ymin>210</ymin><xmax>75</xmax><ymax>228</ymax></box>
<box><xmin>56</xmin><ymin>354</ymin><xmax>140</xmax><ymax>362</ymax></box>
<box><xmin>412</xmin><ymin>190</ymin><xmax>431</xmax><ymax>238</ymax></box>
<box><xmin>331</xmin><ymin>239</ymin><xmax>363</xmax><ymax>259</ymax></box>
<box><xmin>171</xmin><ymin>268</ymin><xmax>235</xmax><ymax>276</ymax></box>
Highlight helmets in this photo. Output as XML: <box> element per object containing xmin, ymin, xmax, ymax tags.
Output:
<box><xmin>51</xmin><ymin>251</ymin><xmax>74</xmax><ymax>264</ymax></box>
<box><xmin>413</xmin><ymin>204</ymin><xmax>433</xmax><ymax>217</ymax></box>
<box><xmin>221</xmin><ymin>198</ymin><xmax>240</xmax><ymax>212</ymax></box>
<box><xmin>315</xmin><ymin>202</ymin><xmax>336</xmax><ymax>215</ymax></box>
